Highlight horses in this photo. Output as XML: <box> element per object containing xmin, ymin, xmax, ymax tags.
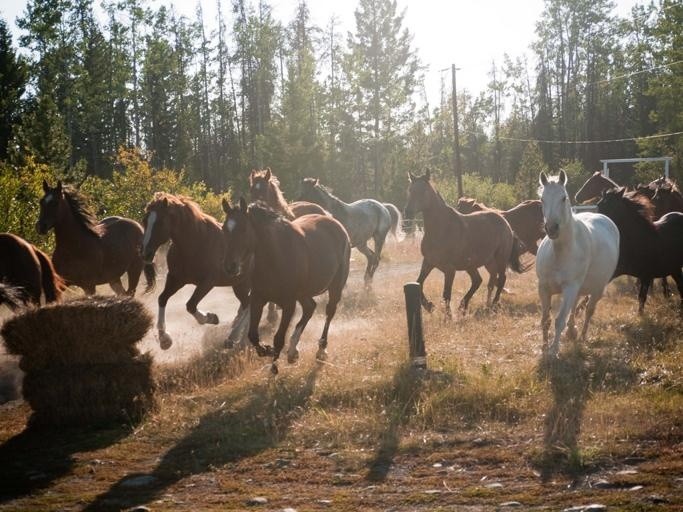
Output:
<box><xmin>2</xmin><ymin>177</ymin><xmax>156</xmax><ymax>313</ymax></box>
<box><xmin>405</xmin><ymin>170</ymin><xmax>680</xmax><ymax>358</ymax></box>
<box><xmin>141</xmin><ymin>168</ymin><xmax>406</xmax><ymax>375</ymax></box>
